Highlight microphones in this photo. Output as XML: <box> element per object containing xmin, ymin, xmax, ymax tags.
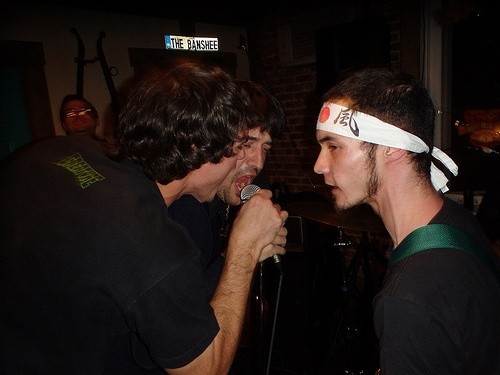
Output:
<box><xmin>240</xmin><ymin>184</ymin><xmax>282</xmax><ymax>264</ymax></box>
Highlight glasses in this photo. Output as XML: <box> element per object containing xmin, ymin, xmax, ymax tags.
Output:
<box><xmin>62</xmin><ymin>107</ymin><xmax>93</xmax><ymax>117</ymax></box>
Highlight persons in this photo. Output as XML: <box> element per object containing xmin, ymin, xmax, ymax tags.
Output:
<box><xmin>60</xmin><ymin>94</ymin><xmax>103</xmax><ymax>144</ymax></box>
<box><xmin>312</xmin><ymin>69</ymin><xmax>499</xmax><ymax>374</ymax></box>
<box><xmin>167</xmin><ymin>74</ymin><xmax>290</xmax><ymax>374</ymax></box>
<box><xmin>0</xmin><ymin>52</ymin><xmax>282</xmax><ymax>374</ymax></box>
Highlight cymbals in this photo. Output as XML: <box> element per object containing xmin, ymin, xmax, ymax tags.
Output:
<box><xmin>285</xmin><ymin>200</ymin><xmax>387</xmax><ymax>233</ymax></box>
